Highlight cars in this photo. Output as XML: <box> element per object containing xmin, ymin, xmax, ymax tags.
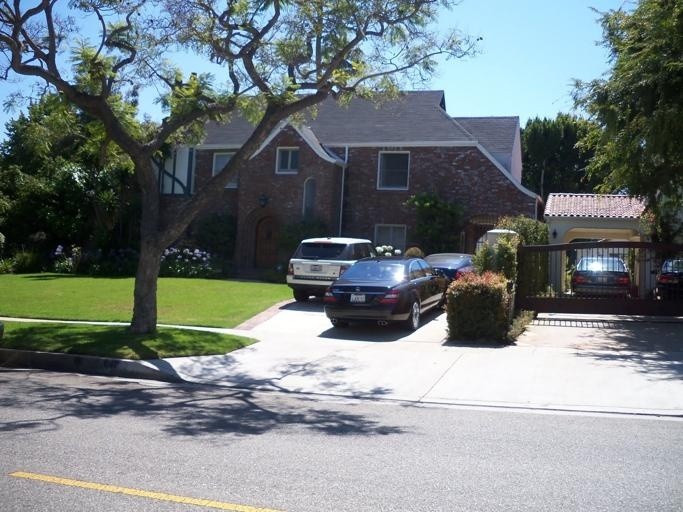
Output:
<box><xmin>569</xmin><ymin>256</ymin><xmax>633</xmax><ymax>298</ymax></box>
<box><xmin>423</xmin><ymin>252</ymin><xmax>473</xmax><ymax>283</ymax></box>
<box><xmin>323</xmin><ymin>256</ymin><xmax>450</xmax><ymax>331</ymax></box>
<box><xmin>649</xmin><ymin>259</ymin><xmax>682</xmax><ymax>303</ymax></box>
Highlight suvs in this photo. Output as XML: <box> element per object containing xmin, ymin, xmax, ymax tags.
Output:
<box><xmin>285</xmin><ymin>237</ymin><xmax>378</xmax><ymax>303</ymax></box>
